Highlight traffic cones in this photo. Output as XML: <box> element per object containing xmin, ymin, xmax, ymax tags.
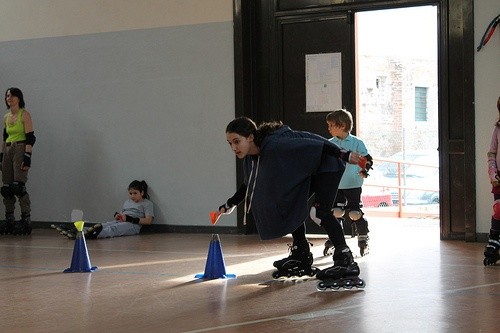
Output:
<box><xmin>194</xmin><ymin>233</ymin><xmax>236</xmax><ymax>280</ymax></box>
<box><xmin>63</xmin><ymin>232</ymin><xmax>99</xmax><ymax>273</ymax></box>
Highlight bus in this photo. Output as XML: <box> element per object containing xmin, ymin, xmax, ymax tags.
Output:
<box><xmin>360</xmin><ymin>149</ymin><xmax>439</xmax><ymax>209</ymax></box>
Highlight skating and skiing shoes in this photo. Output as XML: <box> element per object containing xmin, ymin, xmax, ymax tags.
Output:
<box><xmin>6</xmin><ymin>220</ymin><xmax>33</xmax><ymax>235</ymax></box>
<box><xmin>316</xmin><ymin>251</ymin><xmax>367</xmax><ymax>291</ymax></box>
<box><xmin>0</xmin><ymin>219</ymin><xmax>16</xmax><ymax>235</ymax></box>
<box><xmin>85</xmin><ymin>224</ymin><xmax>104</xmax><ymax>239</ymax></box>
<box><xmin>51</xmin><ymin>224</ymin><xmax>76</xmax><ymax>239</ymax></box>
<box><xmin>356</xmin><ymin>234</ymin><xmax>370</xmax><ymax>256</ymax></box>
<box><xmin>482</xmin><ymin>229</ymin><xmax>500</xmax><ymax>267</ymax></box>
<box><xmin>322</xmin><ymin>239</ymin><xmax>334</xmax><ymax>258</ymax></box>
<box><xmin>271</xmin><ymin>252</ymin><xmax>321</xmax><ymax>280</ymax></box>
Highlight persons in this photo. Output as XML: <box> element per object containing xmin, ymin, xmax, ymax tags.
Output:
<box><xmin>323</xmin><ymin>109</ymin><xmax>373</xmax><ymax>257</ymax></box>
<box><xmin>219</xmin><ymin>117</ymin><xmax>366</xmax><ymax>290</ymax></box>
<box><xmin>0</xmin><ymin>87</ymin><xmax>36</xmax><ymax>235</ymax></box>
<box><xmin>51</xmin><ymin>180</ymin><xmax>154</xmax><ymax>239</ymax></box>
<box><xmin>483</xmin><ymin>96</ymin><xmax>500</xmax><ymax>265</ymax></box>
